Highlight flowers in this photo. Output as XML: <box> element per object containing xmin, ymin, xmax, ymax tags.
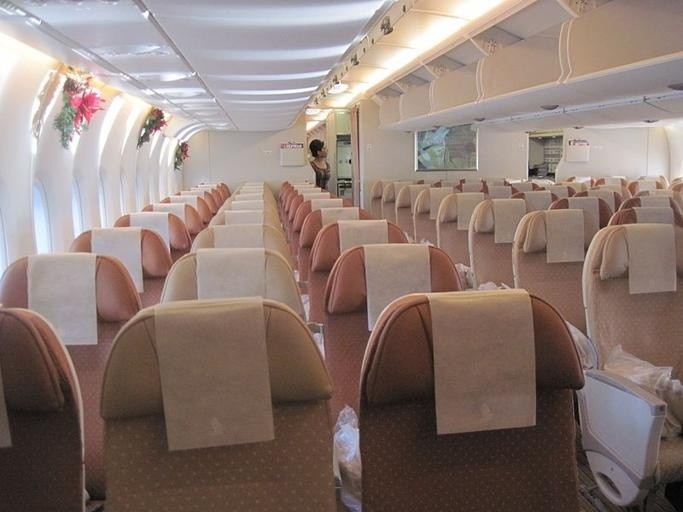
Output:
<box><xmin>173</xmin><ymin>138</ymin><xmax>189</xmax><ymax>171</ymax></box>
<box><xmin>51</xmin><ymin>64</ymin><xmax>112</xmax><ymax>152</ymax></box>
<box><xmin>136</xmin><ymin>106</ymin><xmax>167</xmax><ymax>151</ymax></box>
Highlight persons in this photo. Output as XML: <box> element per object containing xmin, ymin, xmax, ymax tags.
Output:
<box><xmin>305</xmin><ymin>139</ymin><xmax>332</xmax><ymax>193</ymax></box>
